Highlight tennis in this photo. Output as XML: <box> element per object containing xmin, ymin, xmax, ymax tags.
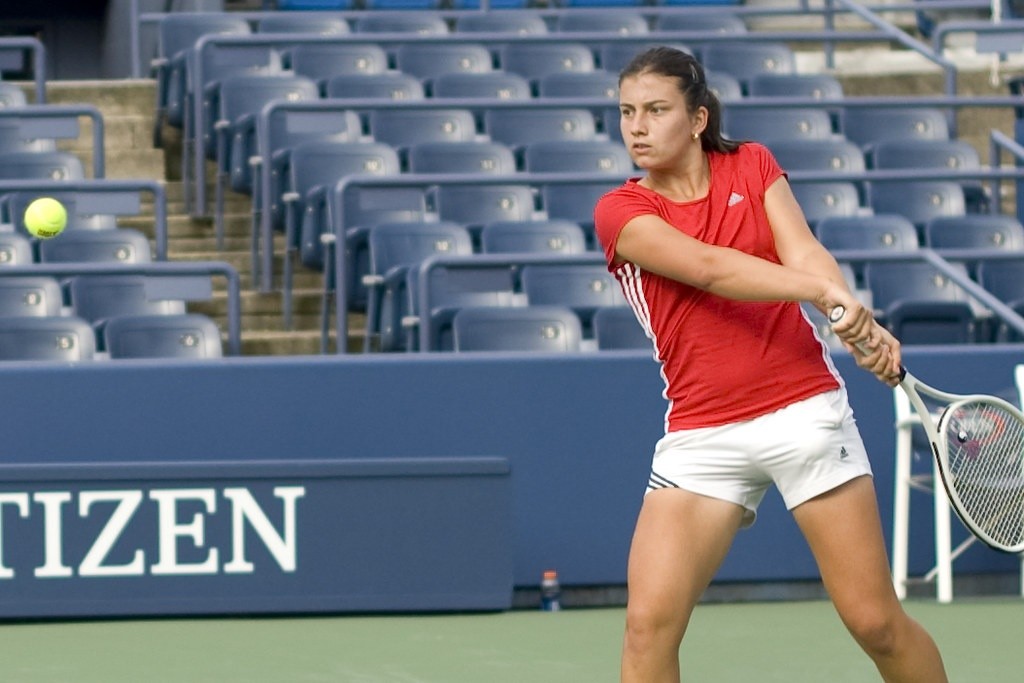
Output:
<box><xmin>23</xmin><ymin>196</ymin><xmax>69</xmax><ymax>240</ymax></box>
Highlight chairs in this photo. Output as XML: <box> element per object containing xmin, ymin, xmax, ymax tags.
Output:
<box><xmin>0</xmin><ymin>5</ymin><xmax>1024</xmax><ymax>362</ymax></box>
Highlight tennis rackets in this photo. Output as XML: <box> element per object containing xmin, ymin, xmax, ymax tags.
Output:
<box><xmin>829</xmin><ymin>304</ymin><xmax>1024</xmax><ymax>556</ymax></box>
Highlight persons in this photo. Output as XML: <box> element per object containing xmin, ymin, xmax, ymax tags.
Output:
<box><xmin>594</xmin><ymin>47</ymin><xmax>949</xmax><ymax>682</ymax></box>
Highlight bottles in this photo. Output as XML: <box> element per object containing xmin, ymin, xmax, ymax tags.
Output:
<box><xmin>540</xmin><ymin>570</ymin><xmax>562</xmax><ymax>612</ymax></box>
